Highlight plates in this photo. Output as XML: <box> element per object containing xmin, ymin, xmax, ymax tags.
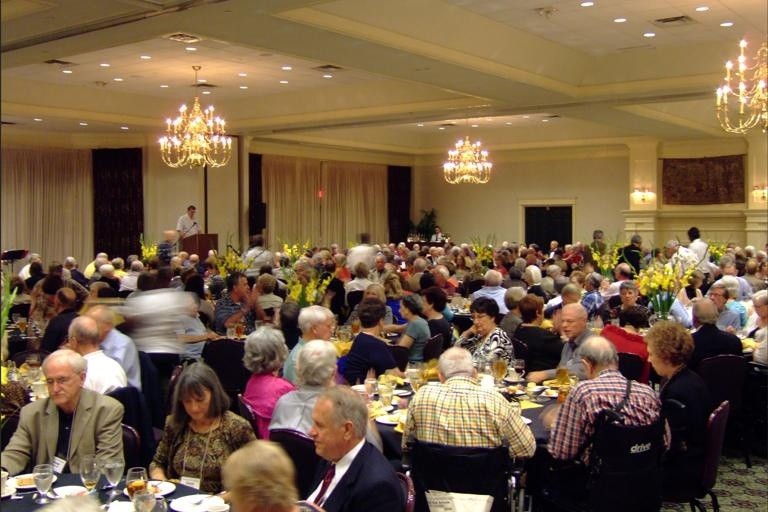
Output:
<box><xmin>0</xmin><ymin>472</ymin><xmax>229</xmax><ymax>512</ymax></box>
<box><xmin>351</xmin><ymin>384</ymin><xmax>559</xmax><ymax>425</ymax></box>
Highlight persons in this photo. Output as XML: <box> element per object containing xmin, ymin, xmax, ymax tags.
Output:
<box><xmin>0</xmin><ymin>227</ymin><xmax>767</xmax><ymax>512</ymax></box>
<box><xmin>176</xmin><ymin>205</ymin><xmax>201</xmax><ymax>239</ymax></box>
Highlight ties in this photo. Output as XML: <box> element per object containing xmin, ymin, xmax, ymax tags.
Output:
<box><xmin>314</xmin><ymin>465</ymin><xmax>335</xmax><ymax>502</ymax></box>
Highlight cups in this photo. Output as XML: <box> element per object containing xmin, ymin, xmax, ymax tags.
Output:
<box><xmin>32</xmin><ymin>383</ymin><xmax>45</xmax><ymax>396</ymax></box>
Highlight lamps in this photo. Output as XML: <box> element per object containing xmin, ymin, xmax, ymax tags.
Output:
<box><xmin>444</xmin><ymin>119</ymin><xmax>492</xmax><ymax>185</ymax></box>
<box><xmin>716</xmin><ymin>40</ymin><xmax>768</xmax><ymax>135</ymax></box>
<box><xmin>160</xmin><ymin>67</ymin><xmax>232</xmax><ymax>169</ymax></box>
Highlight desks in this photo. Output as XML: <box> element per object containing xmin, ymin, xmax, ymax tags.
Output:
<box><xmin>406</xmin><ymin>242</ymin><xmax>444</xmax><ymax>250</ymax></box>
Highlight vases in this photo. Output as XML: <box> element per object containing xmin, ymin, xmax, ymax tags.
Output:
<box><xmin>480</xmin><ymin>266</ymin><xmax>489</xmax><ymax>277</ymax></box>
<box><xmin>715</xmin><ymin>260</ymin><xmax>720</xmax><ymax>266</ymax></box>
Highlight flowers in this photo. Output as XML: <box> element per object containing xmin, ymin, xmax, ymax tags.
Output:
<box><xmin>701</xmin><ymin>238</ymin><xmax>729</xmax><ymax>261</ymax></box>
<box><xmin>212</xmin><ymin>232</ymin><xmax>271</xmax><ymax>289</ymax></box>
<box><xmin>588</xmin><ymin>239</ymin><xmax>622</xmax><ymax>278</ymax></box>
<box><xmin>140</xmin><ymin>233</ymin><xmax>159</xmax><ymax>264</ymax></box>
<box><xmin>471</xmin><ymin>234</ymin><xmax>497</xmax><ymax>275</ymax></box>
<box><xmin>281</xmin><ymin>238</ymin><xmax>310</xmax><ymax>267</ymax></box>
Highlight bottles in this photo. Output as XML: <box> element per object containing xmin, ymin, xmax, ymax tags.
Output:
<box><xmin>227</xmin><ymin>324</ymin><xmax>235</xmax><ymax>339</ymax></box>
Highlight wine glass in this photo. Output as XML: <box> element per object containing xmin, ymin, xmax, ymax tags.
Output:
<box><xmin>365</xmin><ymin>359</ymin><xmax>571</xmax><ymax>407</ymax></box>
<box><xmin>235</xmin><ymin>324</ymin><xmax>243</xmax><ymax>337</ymax></box>
<box><xmin>255</xmin><ymin>320</ymin><xmax>263</xmax><ymax>330</ymax></box>
<box><xmin>32</xmin><ymin>456</ymin><xmax>167</xmax><ymax>512</ymax></box>
<box><xmin>29</xmin><ymin>354</ymin><xmax>41</xmax><ymax>371</ymax></box>
<box><xmin>11</xmin><ymin>313</ymin><xmax>48</xmax><ymax>337</ymax></box>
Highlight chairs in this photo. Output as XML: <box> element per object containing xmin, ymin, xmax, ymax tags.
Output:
<box><xmin>0</xmin><ymin>249</ymin><xmax>27</xmax><ymax>279</ymax></box>
